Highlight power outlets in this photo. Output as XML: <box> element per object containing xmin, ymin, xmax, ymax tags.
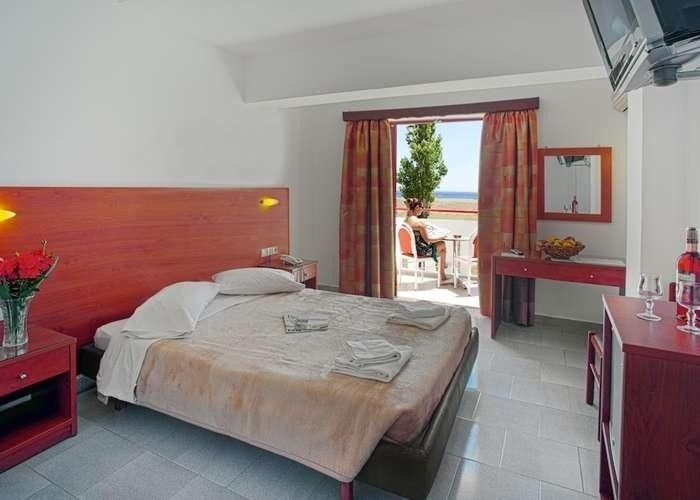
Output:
<box><xmin>261</xmin><ymin>248</ymin><xmax>268</xmax><ymax>258</ymax></box>
<box><xmin>274</xmin><ymin>246</ymin><xmax>279</xmax><ymax>255</ymax></box>
<box><xmin>268</xmin><ymin>247</ymin><xmax>273</xmax><ymax>255</ymax></box>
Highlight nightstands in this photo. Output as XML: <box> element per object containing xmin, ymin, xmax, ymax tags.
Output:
<box><xmin>259</xmin><ymin>258</ymin><xmax>318</xmax><ymax>291</ymax></box>
<box><xmin>1</xmin><ymin>323</ymin><xmax>76</xmax><ymax>472</ymax></box>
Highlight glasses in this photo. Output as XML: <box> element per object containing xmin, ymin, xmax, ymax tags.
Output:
<box><xmin>419</xmin><ymin>205</ymin><xmax>424</xmax><ymax>209</ymax></box>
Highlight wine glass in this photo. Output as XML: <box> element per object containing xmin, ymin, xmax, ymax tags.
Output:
<box><xmin>634</xmin><ymin>272</ymin><xmax>663</xmax><ymax>322</ymax></box>
<box><xmin>677</xmin><ymin>280</ymin><xmax>700</xmax><ymax>334</ymax></box>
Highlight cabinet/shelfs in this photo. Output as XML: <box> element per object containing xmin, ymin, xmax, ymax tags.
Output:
<box><xmin>596</xmin><ymin>292</ymin><xmax>700</xmax><ymax>499</ymax></box>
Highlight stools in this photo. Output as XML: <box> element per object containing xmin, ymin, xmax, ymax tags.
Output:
<box><xmin>586</xmin><ymin>330</ymin><xmax>606</xmax><ymax>406</ymax></box>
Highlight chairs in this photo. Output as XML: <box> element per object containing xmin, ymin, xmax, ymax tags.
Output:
<box><xmin>454</xmin><ymin>227</ymin><xmax>482</xmax><ymax>294</ymax></box>
<box><xmin>399</xmin><ymin>223</ymin><xmax>440</xmax><ymax>289</ymax></box>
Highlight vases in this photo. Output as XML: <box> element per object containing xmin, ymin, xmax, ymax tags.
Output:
<box><xmin>1</xmin><ymin>295</ymin><xmax>34</xmax><ymax>347</ymax></box>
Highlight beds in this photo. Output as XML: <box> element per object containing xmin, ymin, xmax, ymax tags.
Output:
<box><xmin>77</xmin><ymin>275</ymin><xmax>480</xmax><ymax>499</ymax></box>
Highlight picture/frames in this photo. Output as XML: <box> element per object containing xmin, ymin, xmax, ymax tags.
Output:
<box><xmin>536</xmin><ymin>145</ymin><xmax>613</xmax><ymax>223</ymax></box>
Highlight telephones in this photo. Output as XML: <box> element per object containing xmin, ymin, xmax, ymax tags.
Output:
<box><xmin>280</xmin><ymin>253</ymin><xmax>302</xmax><ymax>267</ymax></box>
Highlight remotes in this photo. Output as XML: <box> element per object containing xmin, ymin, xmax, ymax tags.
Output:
<box><xmin>509</xmin><ymin>249</ymin><xmax>523</xmax><ymax>256</ymax></box>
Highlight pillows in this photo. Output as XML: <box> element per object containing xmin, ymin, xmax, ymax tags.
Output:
<box><xmin>212</xmin><ymin>267</ymin><xmax>304</xmax><ymax>296</ymax></box>
<box><xmin>121</xmin><ymin>281</ymin><xmax>221</xmax><ymax>340</ymax></box>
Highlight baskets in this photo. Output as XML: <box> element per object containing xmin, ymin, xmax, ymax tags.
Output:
<box><xmin>535</xmin><ymin>240</ymin><xmax>586</xmax><ymax>259</ymax></box>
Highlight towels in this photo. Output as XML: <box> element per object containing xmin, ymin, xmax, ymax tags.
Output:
<box><xmin>327</xmin><ymin>344</ymin><xmax>415</xmax><ymax>383</ymax></box>
<box><xmin>398</xmin><ymin>300</ymin><xmax>444</xmax><ymax>319</ymax></box>
<box><xmin>384</xmin><ymin>304</ymin><xmax>450</xmax><ymax>331</ymax></box>
<box><xmin>343</xmin><ymin>338</ymin><xmax>401</xmax><ymax>366</ymax></box>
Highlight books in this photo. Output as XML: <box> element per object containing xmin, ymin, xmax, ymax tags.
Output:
<box><xmin>283</xmin><ymin>310</ymin><xmax>329</xmax><ymax>333</ymax></box>
<box><xmin>426</xmin><ymin>223</ymin><xmax>451</xmax><ymax>237</ymax></box>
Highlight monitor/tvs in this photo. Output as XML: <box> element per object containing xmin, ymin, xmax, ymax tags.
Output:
<box><xmin>581</xmin><ymin>0</ymin><xmax>700</xmax><ymax>98</ymax></box>
<box><xmin>558</xmin><ymin>155</ymin><xmax>592</xmax><ymax>168</ymax></box>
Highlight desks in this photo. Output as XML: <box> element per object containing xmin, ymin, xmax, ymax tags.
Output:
<box><xmin>428</xmin><ymin>233</ymin><xmax>471</xmax><ymax>289</ymax></box>
<box><xmin>488</xmin><ymin>247</ymin><xmax>626</xmax><ymax>338</ymax></box>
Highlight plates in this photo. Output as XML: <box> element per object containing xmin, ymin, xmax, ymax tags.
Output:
<box><xmin>538</xmin><ymin>244</ymin><xmax>585</xmax><ymax>260</ymax></box>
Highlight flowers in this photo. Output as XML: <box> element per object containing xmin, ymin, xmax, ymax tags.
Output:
<box><xmin>0</xmin><ymin>239</ymin><xmax>59</xmax><ymax>335</ymax></box>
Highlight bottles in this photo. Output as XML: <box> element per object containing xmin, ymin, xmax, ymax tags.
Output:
<box><xmin>572</xmin><ymin>195</ymin><xmax>578</xmax><ymax>214</ymax></box>
<box><xmin>676</xmin><ymin>226</ymin><xmax>700</xmax><ymax>323</ymax></box>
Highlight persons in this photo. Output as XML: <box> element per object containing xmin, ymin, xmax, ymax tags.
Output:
<box><xmin>404</xmin><ymin>197</ymin><xmax>448</xmax><ymax>280</ymax></box>
